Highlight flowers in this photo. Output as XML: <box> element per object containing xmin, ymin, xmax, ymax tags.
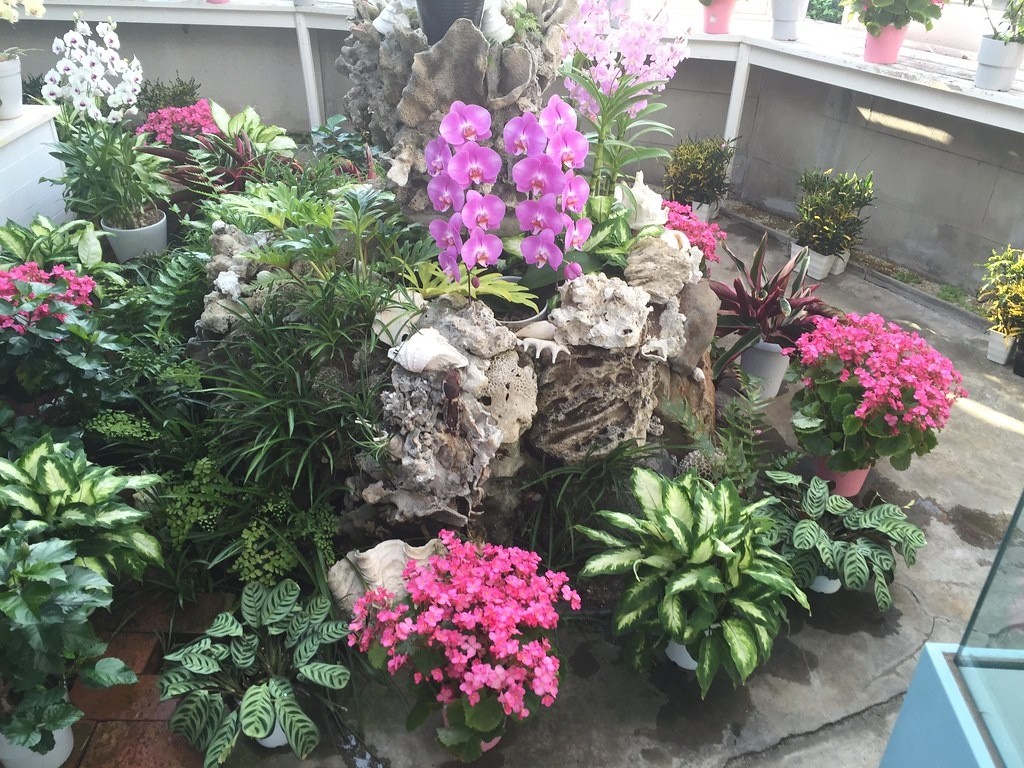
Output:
<box><xmin>791</xmin><ymin>165</ymin><xmax>881</xmax><ymax>262</ymax></box>
<box><xmin>347</xmin><ymin>529</ymin><xmax>581</xmax><ymax>763</ymax></box>
<box><xmin>0</xmin><ymin>0</ymin><xmax>226</xmax><ymax>149</ymax></box>
<box><xmin>0</xmin><ymin>263</ymin><xmax>98</xmax><ymax>344</ymax></box>
<box><xmin>976</xmin><ymin>242</ymin><xmax>1024</xmax><ymax>351</ymax></box>
<box><xmin>780</xmin><ymin>312</ymin><xmax>968</xmax><ymax>471</ymax></box>
<box><xmin>420</xmin><ymin>0</ymin><xmax>744</xmax><ymax>307</ymax></box>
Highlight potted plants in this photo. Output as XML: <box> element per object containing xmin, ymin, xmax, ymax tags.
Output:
<box><xmin>309</xmin><ymin>114</ymin><xmax>378</xmax><ymax>181</ymax></box>
<box><xmin>574</xmin><ymin>462</ymin><xmax>812</xmax><ymax>701</ymax></box>
<box><xmin>699</xmin><ymin>0</ymin><xmax>736</xmax><ymax>34</ymax></box>
<box><xmin>840</xmin><ymin>0</ymin><xmax>949</xmax><ymax>64</ymax></box>
<box><xmin>0</xmin><ymin>426</ymin><xmax>348</xmax><ymax>768</ymax></box>
<box><xmin>770</xmin><ymin>471</ymin><xmax>926</xmax><ymax>614</ymax></box>
<box><xmin>965</xmin><ymin>0</ymin><xmax>1024</xmax><ymax>90</ymax></box>
<box><xmin>38</xmin><ymin>117</ymin><xmax>176</xmax><ymax>260</ymax></box>
<box><xmin>714</xmin><ymin>232</ymin><xmax>835</xmax><ymax>399</ymax></box>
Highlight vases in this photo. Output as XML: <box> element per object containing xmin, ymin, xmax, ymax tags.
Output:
<box><xmin>772</xmin><ymin>0</ymin><xmax>800</xmax><ymax>41</ymax></box>
<box><xmin>809</xmin><ymin>450</ymin><xmax>872</xmax><ymax>498</ymax></box>
<box><xmin>986</xmin><ymin>326</ymin><xmax>1016</xmax><ymax>364</ymax></box>
<box><xmin>437</xmin><ymin>698</ymin><xmax>505</xmax><ymax>751</ymax></box>
<box><xmin>808</xmin><ymin>248</ymin><xmax>835</xmax><ymax>281</ymax></box>
<box><xmin>692</xmin><ymin>202</ymin><xmax>710</xmax><ymax>224</ymax></box>
<box><xmin>790</xmin><ymin>240</ymin><xmax>808</xmax><ymax>274</ymax></box>
<box><xmin>496</xmin><ymin>298</ymin><xmax>549</xmax><ymax>330</ymax></box>
<box><xmin>710</xmin><ymin>201</ymin><xmax>721</xmax><ymax>219</ymax></box>
<box><xmin>0</xmin><ymin>59</ymin><xmax>22</xmax><ymax>120</ymax></box>
<box><xmin>829</xmin><ymin>250</ymin><xmax>851</xmax><ymax>275</ymax></box>
<box><xmin>1014</xmin><ymin>343</ymin><xmax>1024</xmax><ymax>378</ymax></box>
